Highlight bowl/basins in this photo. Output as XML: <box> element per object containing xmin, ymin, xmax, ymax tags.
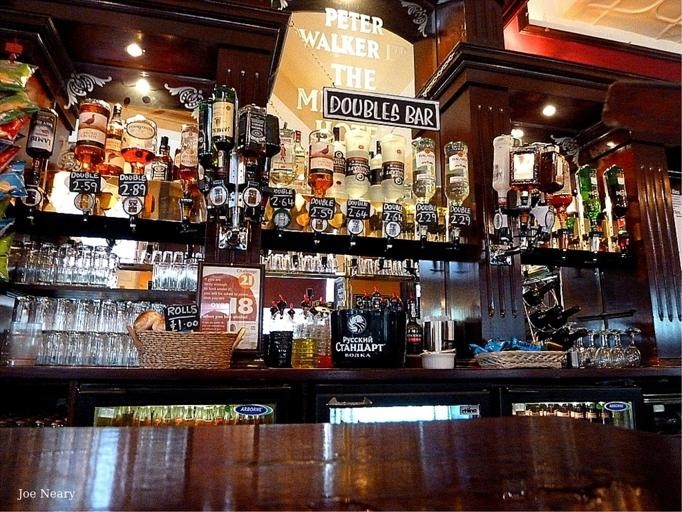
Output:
<box><xmin>421</xmin><ymin>353</ymin><xmax>456</xmax><ymax>370</ymax></box>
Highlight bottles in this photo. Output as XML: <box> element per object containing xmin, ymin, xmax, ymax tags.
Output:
<box><xmin>524</xmin><ymin>271</ymin><xmax>586</xmax><ymax>352</ymax></box>
<box><xmin>25</xmin><ymin>79</ymin><xmax>629</xmax><ymax>259</ymax></box>
<box><xmin>266</xmin><ymin>287</ymin><xmax>332</xmax><ymax>368</ymax></box>
<box><xmin>407</xmin><ymin>298</ymin><xmax>423</xmax><ymax>353</ymax></box>
<box><xmin>512</xmin><ymin>401</ymin><xmax>612</xmax><ymax>424</ymax></box>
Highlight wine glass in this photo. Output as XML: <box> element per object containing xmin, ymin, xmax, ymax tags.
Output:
<box><xmin>574</xmin><ymin>327</ymin><xmax>641</xmax><ymax>368</ymax></box>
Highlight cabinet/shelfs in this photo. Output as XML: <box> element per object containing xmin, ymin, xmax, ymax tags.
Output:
<box><xmin>0</xmin><ymin>39</ymin><xmax>682</xmax><ymax>387</ymax></box>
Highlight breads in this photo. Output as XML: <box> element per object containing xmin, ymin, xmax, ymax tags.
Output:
<box><xmin>133</xmin><ymin>310</ymin><xmax>165</xmax><ymax>331</ymax></box>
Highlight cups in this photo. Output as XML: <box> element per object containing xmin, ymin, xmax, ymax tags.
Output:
<box><xmin>261</xmin><ymin>249</ymin><xmax>419</xmax><ymax>277</ymax></box>
<box><xmin>7</xmin><ymin>295</ymin><xmax>167</xmax><ymax>367</ymax></box>
<box><xmin>19</xmin><ymin>240</ymin><xmax>203</xmax><ymax>291</ymax></box>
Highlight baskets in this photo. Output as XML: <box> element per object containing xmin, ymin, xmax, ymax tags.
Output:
<box><xmin>125</xmin><ymin>325</ymin><xmax>247</xmax><ymax>369</ymax></box>
<box><xmin>474</xmin><ymin>349</ymin><xmax>568</xmax><ymax>370</ymax></box>
